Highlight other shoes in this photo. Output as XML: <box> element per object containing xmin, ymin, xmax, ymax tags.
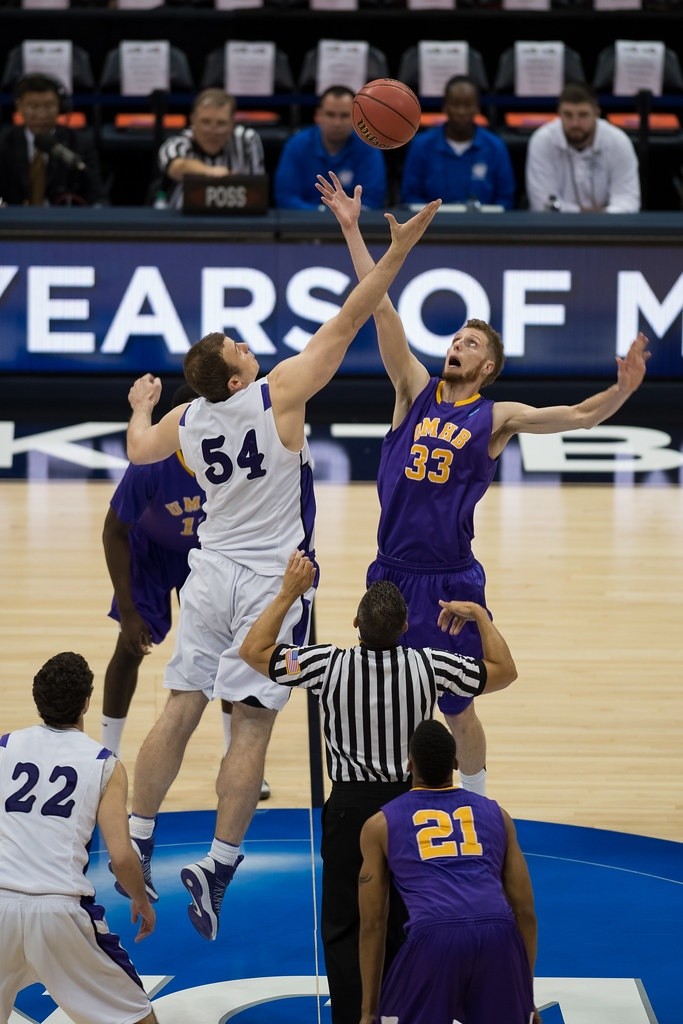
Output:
<box><xmin>260</xmin><ymin>779</ymin><xmax>271</xmax><ymax>799</ymax></box>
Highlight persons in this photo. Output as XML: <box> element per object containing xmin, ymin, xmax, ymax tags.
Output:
<box><xmin>101</xmin><ymin>196</ymin><xmax>442</xmax><ymax>939</ymax></box>
<box><xmin>241</xmin><ymin>555</ymin><xmax>536</xmax><ymax>1024</ymax></box>
<box><xmin>0</xmin><ymin>73</ymin><xmax>643</xmax><ymax>216</ymax></box>
<box><xmin>313</xmin><ymin>173</ymin><xmax>649</xmax><ymax>791</ymax></box>
<box><xmin>0</xmin><ymin>652</ymin><xmax>160</xmax><ymax>1024</ymax></box>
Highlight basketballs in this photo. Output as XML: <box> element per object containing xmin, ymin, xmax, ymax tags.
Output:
<box><xmin>350</xmin><ymin>77</ymin><xmax>422</xmax><ymax>148</ymax></box>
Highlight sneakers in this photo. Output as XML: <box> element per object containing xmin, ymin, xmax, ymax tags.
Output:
<box><xmin>108</xmin><ymin>834</ymin><xmax>159</xmax><ymax>903</ymax></box>
<box><xmin>181</xmin><ymin>855</ymin><xmax>244</xmax><ymax>941</ymax></box>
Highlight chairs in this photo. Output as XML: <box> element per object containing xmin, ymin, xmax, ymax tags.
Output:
<box><xmin>392</xmin><ymin>42</ymin><xmax>493</xmax><ymax>127</ymax></box>
<box><xmin>590</xmin><ymin>41</ymin><xmax>683</xmax><ymax>132</ymax></box>
<box><xmin>0</xmin><ymin>41</ymin><xmax>96</xmax><ymax>127</ymax></box>
<box><xmin>96</xmin><ymin>39</ymin><xmax>196</xmax><ymax>128</ymax></box>
<box><xmin>493</xmin><ymin>42</ymin><xmax>586</xmax><ymax>128</ymax></box>
<box><xmin>297</xmin><ymin>40</ymin><xmax>389</xmax><ymax>123</ymax></box>
<box><xmin>203</xmin><ymin>40</ymin><xmax>295</xmax><ymax>124</ymax></box>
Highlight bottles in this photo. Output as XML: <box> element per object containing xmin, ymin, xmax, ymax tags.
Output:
<box><xmin>466</xmin><ymin>195</ymin><xmax>482</xmax><ymax>214</ymax></box>
<box><xmin>545</xmin><ymin>193</ymin><xmax>561</xmax><ymax>213</ymax></box>
<box><xmin>153</xmin><ymin>191</ymin><xmax>168</xmax><ymax>209</ymax></box>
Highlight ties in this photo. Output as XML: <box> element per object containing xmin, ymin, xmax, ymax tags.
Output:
<box><xmin>31</xmin><ymin>149</ymin><xmax>46</xmax><ymax>206</ymax></box>
